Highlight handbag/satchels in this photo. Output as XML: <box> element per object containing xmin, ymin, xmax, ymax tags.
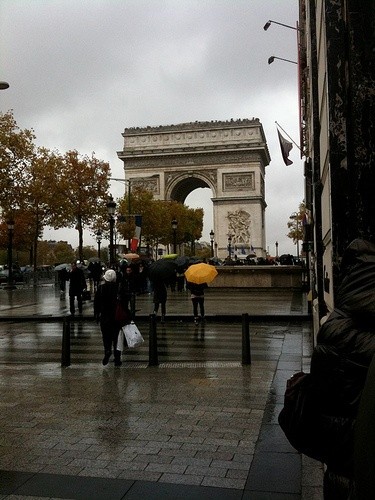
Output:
<box><xmin>82</xmin><ymin>290</ymin><xmax>91</xmax><ymax>300</ymax></box>
<box><xmin>114</xmin><ymin>294</ymin><xmax>127</xmax><ymax>321</ymax></box>
<box><xmin>279</xmin><ymin>373</ymin><xmax>363</xmax><ymax>472</ymax></box>
<box><xmin>116</xmin><ymin>323</ymin><xmax>145</xmax><ymax>351</ymax></box>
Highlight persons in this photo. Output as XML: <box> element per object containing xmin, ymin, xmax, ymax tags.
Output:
<box><xmin>57</xmin><ymin>254</ymin><xmax>293</xmax><ymax>367</ymax></box>
<box><xmin>310</xmin><ymin>240</ymin><xmax>375</xmax><ymax>500</ymax></box>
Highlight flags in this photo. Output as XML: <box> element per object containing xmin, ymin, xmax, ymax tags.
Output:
<box><xmin>277</xmin><ymin>128</ymin><xmax>293</xmax><ymax>166</ymax></box>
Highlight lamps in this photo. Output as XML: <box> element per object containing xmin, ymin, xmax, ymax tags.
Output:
<box><xmin>267</xmin><ymin>56</ymin><xmax>298</xmax><ymax>65</ymax></box>
<box><xmin>264</xmin><ymin>20</ymin><xmax>304</xmax><ymax>34</ymax></box>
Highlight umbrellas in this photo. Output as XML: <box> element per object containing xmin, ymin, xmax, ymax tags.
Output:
<box><xmin>53</xmin><ymin>252</ymin><xmax>288</xmax><ymax>284</ymax></box>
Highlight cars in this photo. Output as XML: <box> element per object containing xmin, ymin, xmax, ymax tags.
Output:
<box><xmin>0</xmin><ymin>264</ymin><xmax>53</xmax><ymax>282</ymax></box>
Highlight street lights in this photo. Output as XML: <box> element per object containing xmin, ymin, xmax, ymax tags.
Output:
<box><xmin>275</xmin><ymin>241</ymin><xmax>278</xmax><ymax>256</ymax></box>
<box><xmin>171</xmin><ymin>217</ymin><xmax>178</xmax><ymax>253</ymax></box>
<box><xmin>210</xmin><ymin>229</ymin><xmax>214</xmax><ymax>257</ymax></box>
<box><xmin>97</xmin><ymin>229</ymin><xmax>102</xmax><ymax>259</ymax></box>
<box><xmin>228</xmin><ymin>235</ymin><xmax>232</xmax><ymax>258</ymax></box>
<box><xmin>106</xmin><ymin>193</ymin><xmax>116</xmax><ymax>269</ymax></box>
<box><xmin>5</xmin><ymin>215</ymin><xmax>16</xmax><ymax>289</ymax></box>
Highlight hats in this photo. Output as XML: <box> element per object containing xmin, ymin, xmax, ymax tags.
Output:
<box><xmin>103</xmin><ymin>269</ymin><xmax>116</xmax><ymax>282</ymax></box>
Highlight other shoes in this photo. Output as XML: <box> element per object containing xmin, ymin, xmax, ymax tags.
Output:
<box><xmin>61</xmin><ymin>290</ymin><xmax>64</xmax><ymax>295</ymax></box>
<box><xmin>71</xmin><ymin>313</ymin><xmax>74</xmax><ymax>316</ymax></box>
<box><xmin>195</xmin><ymin>321</ymin><xmax>198</xmax><ymax>325</ymax></box>
<box><xmin>201</xmin><ymin>317</ymin><xmax>207</xmax><ymax>322</ymax></box>
<box><xmin>115</xmin><ymin>360</ymin><xmax>121</xmax><ymax>366</ymax></box>
<box><xmin>154</xmin><ymin>311</ymin><xmax>157</xmax><ymax>318</ymax></box>
<box><xmin>103</xmin><ymin>351</ymin><xmax>112</xmax><ymax>366</ymax></box>
<box><xmin>79</xmin><ymin>309</ymin><xmax>82</xmax><ymax>314</ymax></box>
<box><xmin>161</xmin><ymin>320</ymin><xmax>164</xmax><ymax>324</ymax></box>
